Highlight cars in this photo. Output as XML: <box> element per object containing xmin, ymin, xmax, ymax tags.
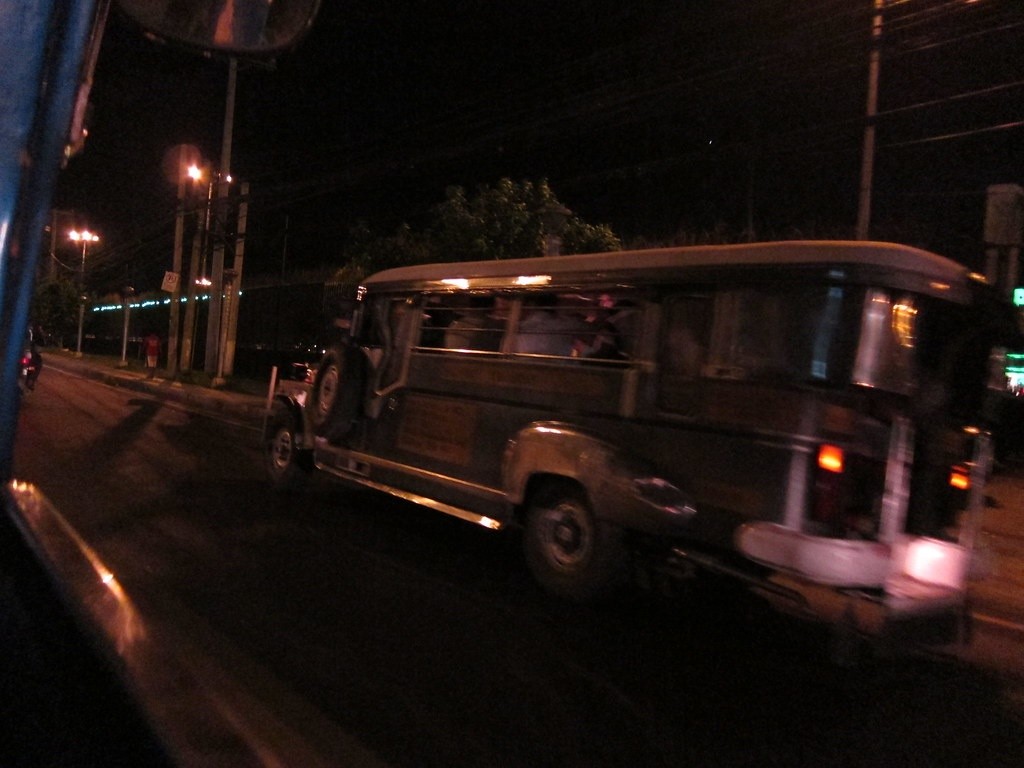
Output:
<box><xmin>19</xmin><ymin>324</ymin><xmax>45</xmax><ymax>389</ymax></box>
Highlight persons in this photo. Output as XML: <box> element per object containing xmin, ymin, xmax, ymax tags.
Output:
<box><xmin>143</xmin><ymin>329</ymin><xmax>160</xmax><ymax>379</ymax></box>
<box><xmin>418</xmin><ymin>291</ymin><xmax>635</xmax><ymax>366</ymax></box>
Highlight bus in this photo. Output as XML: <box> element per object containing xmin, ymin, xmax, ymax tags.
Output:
<box><xmin>761</xmin><ymin>454</ymin><xmax>771</xmax><ymax>473</ymax></box>
<box><xmin>259</xmin><ymin>237</ymin><xmax>1001</xmax><ymax>638</ymax></box>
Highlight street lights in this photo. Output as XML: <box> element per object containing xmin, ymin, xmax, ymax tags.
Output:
<box><xmin>65</xmin><ymin>231</ymin><xmax>102</xmax><ymax>358</ymax></box>
<box><xmin>187</xmin><ymin>161</ymin><xmax>251</xmax><ymax>379</ymax></box>
